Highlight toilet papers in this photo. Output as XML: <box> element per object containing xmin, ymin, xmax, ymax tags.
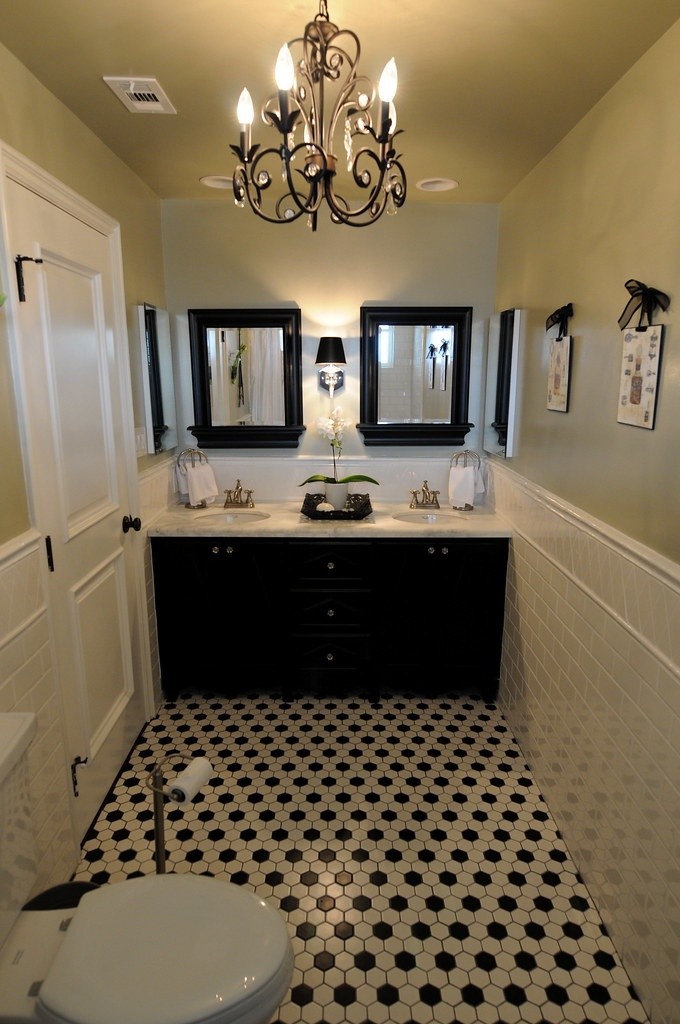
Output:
<box><xmin>167</xmin><ymin>757</ymin><xmax>214</xmax><ymax>805</ymax></box>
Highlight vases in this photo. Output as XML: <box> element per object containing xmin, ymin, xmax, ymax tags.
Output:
<box><xmin>325</xmin><ymin>483</ymin><xmax>348</xmax><ymax>510</ymax></box>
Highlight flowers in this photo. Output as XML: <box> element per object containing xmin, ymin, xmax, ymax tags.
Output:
<box><xmin>297</xmin><ymin>406</ymin><xmax>380</xmax><ymax>487</ymax></box>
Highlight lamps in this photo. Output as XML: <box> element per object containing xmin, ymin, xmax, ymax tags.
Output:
<box><xmin>315</xmin><ymin>337</ymin><xmax>347</xmax><ymax>398</ymax></box>
<box><xmin>229</xmin><ymin>0</ymin><xmax>407</xmax><ymax>231</ymax></box>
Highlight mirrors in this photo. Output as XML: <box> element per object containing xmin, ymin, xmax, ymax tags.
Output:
<box><xmin>186</xmin><ymin>309</ymin><xmax>306</xmax><ymax>449</ymax></box>
<box><xmin>356</xmin><ymin>307</ymin><xmax>475</xmax><ymax>446</ymax></box>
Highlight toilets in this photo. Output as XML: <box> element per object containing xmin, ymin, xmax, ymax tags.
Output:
<box><xmin>0</xmin><ymin>869</ymin><xmax>297</xmax><ymax>1023</ymax></box>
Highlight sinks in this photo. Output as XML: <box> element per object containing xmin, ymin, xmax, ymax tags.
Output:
<box><xmin>194</xmin><ymin>510</ymin><xmax>271</xmax><ymax>526</ymax></box>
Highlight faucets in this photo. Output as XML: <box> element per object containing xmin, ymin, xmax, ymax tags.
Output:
<box><xmin>392</xmin><ymin>510</ymin><xmax>469</xmax><ymax>525</ymax></box>
<box><xmin>421</xmin><ymin>479</ymin><xmax>431</xmax><ymax>504</ymax></box>
<box><xmin>233</xmin><ymin>478</ymin><xmax>244</xmax><ymax>503</ymax></box>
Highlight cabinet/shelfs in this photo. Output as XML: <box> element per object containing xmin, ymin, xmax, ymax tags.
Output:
<box><xmin>151</xmin><ymin>536</ymin><xmax>509</xmax><ymax>704</ymax></box>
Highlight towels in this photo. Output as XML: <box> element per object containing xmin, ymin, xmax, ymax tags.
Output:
<box><xmin>448</xmin><ymin>465</ymin><xmax>486</xmax><ymax>508</ymax></box>
<box><xmin>171</xmin><ymin>462</ymin><xmax>219</xmax><ymax>506</ymax></box>
